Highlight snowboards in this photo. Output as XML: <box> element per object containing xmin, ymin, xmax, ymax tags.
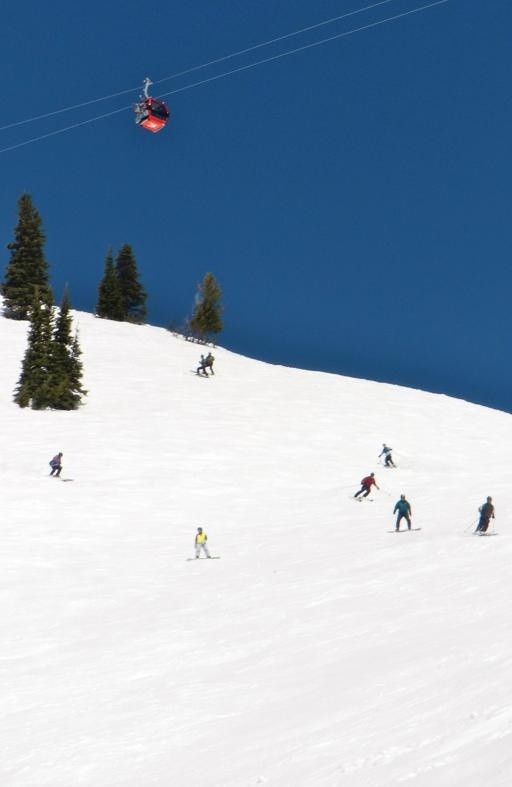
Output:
<box><xmin>350</xmin><ymin>497</ymin><xmax>375</xmax><ymax>502</ymax></box>
<box><xmin>471</xmin><ymin>532</ymin><xmax>498</xmax><ymax>536</ymax></box>
<box><xmin>388</xmin><ymin>528</ymin><xmax>420</xmax><ymax>533</ymax></box>
<box><xmin>380</xmin><ymin>465</ymin><xmax>398</xmax><ymax>468</ymax></box>
<box><xmin>46</xmin><ymin>474</ymin><xmax>74</xmax><ymax>482</ymax></box>
<box><xmin>190</xmin><ymin>370</ymin><xmax>210</xmax><ymax>378</ymax></box>
<box><xmin>187</xmin><ymin>557</ymin><xmax>220</xmax><ymax>560</ymax></box>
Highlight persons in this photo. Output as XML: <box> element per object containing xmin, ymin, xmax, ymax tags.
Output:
<box><xmin>197</xmin><ymin>355</ymin><xmax>209</xmax><ymax>376</ymax></box>
<box><xmin>393</xmin><ymin>495</ymin><xmax>412</xmax><ymax>532</ymax></box>
<box><xmin>203</xmin><ymin>352</ymin><xmax>215</xmax><ymax>375</ymax></box>
<box><xmin>194</xmin><ymin>528</ymin><xmax>211</xmax><ymax>559</ymax></box>
<box><xmin>475</xmin><ymin>496</ymin><xmax>495</xmax><ymax>532</ymax></box>
<box><xmin>354</xmin><ymin>473</ymin><xmax>380</xmax><ymax>497</ymax></box>
<box><xmin>378</xmin><ymin>444</ymin><xmax>395</xmax><ymax>466</ymax></box>
<box><xmin>49</xmin><ymin>452</ymin><xmax>63</xmax><ymax>477</ymax></box>
